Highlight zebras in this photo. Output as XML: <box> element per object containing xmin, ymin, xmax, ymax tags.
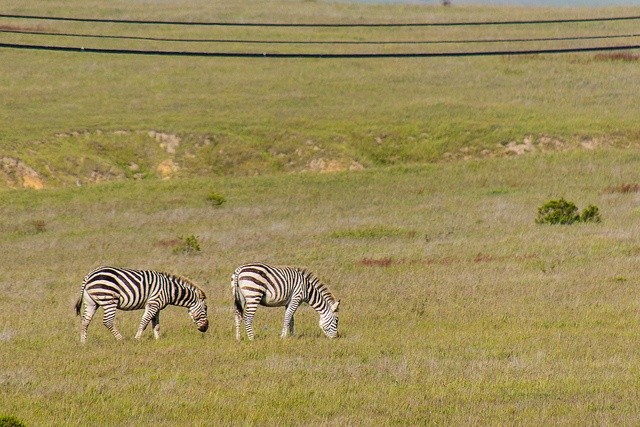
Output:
<box><xmin>231</xmin><ymin>261</ymin><xmax>341</xmax><ymax>341</ymax></box>
<box><xmin>74</xmin><ymin>265</ymin><xmax>209</xmax><ymax>343</ymax></box>
